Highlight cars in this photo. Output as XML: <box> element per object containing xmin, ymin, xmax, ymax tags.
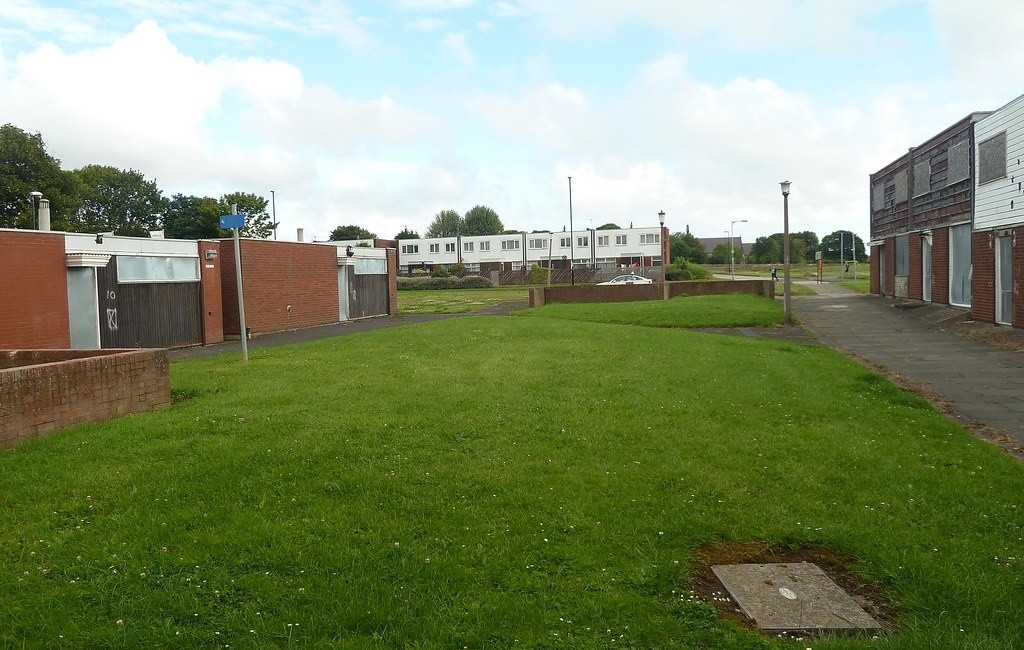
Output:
<box><xmin>596</xmin><ymin>274</ymin><xmax>652</xmax><ymax>285</ymax></box>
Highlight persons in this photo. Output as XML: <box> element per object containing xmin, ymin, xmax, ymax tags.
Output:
<box><xmin>771</xmin><ymin>265</ymin><xmax>778</xmax><ymax>282</ymax></box>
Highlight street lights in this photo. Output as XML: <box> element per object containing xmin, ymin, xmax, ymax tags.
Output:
<box><xmin>270</xmin><ymin>191</ymin><xmax>276</xmax><ymax>239</ymax></box>
<box><xmin>841</xmin><ymin>231</ymin><xmax>848</xmax><ymax>281</ymax></box>
<box><xmin>731</xmin><ymin>220</ymin><xmax>747</xmax><ymax>279</ymax></box>
<box><xmin>568</xmin><ymin>177</ymin><xmax>574</xmax><ymax>285</ymax></box>
<box><xmin>724</xmin><ymin>231</ymin><xmax>730</xmax><ymax>273</ymax></box>
<box><xmin>852</xmin><ymin>232</ymin><xmax>856</xmax><ymax>281</ymax></box>
<box><xmin>658</xmin><ymin>210</ymin><xmax>666</xmax><ymax>282</ymax></box>
<box><xmin>779</xmin><ymin>181</ymin><xmax>794</xmax><ymax>325</ymax></box>
<box><xmin>30</xmin><ymin>191</ymin><xmax>43</xmax><ymax>230</ymax></box>
